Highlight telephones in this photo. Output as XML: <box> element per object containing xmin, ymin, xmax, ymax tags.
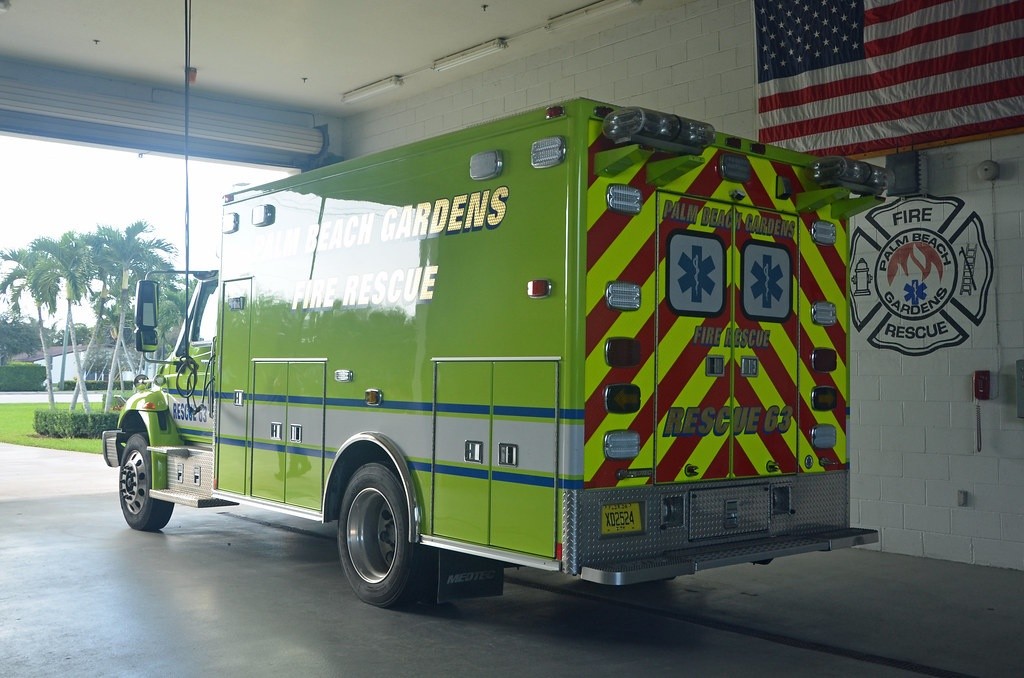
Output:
<box><xmin>971</xmin><ymin>370</ymin><xmax>991</xmax><ymax>402</ymax></box>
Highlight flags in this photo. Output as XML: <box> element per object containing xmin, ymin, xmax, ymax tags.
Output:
<box><xmin>751</xmin><ymin>0</ymin><xmax>1024</xmax><ymax>160</ymax></box>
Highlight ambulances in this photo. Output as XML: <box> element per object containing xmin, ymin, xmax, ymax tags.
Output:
<box><xmin>102</xmin><ymin>94</ymin><xmax>894</xmax><ymax>610</ymax></box>
